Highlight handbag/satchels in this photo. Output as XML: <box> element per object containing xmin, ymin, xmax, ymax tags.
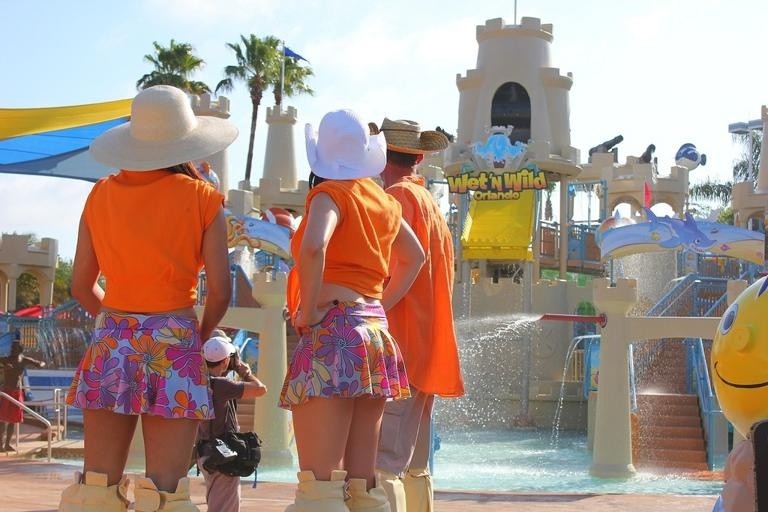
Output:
<box><xmin>194</xmin><ymin>431</ymin><xmax>262</xmax><ymax>478</ymax></box>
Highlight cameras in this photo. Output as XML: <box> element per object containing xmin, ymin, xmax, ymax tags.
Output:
<box><xmin>227</xmin><ymin>354</ymin><xmax>234</xmax><ymax>370</ymax></box>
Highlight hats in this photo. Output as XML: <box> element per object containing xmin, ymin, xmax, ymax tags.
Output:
<box><xmin>368</xmin><ymin>117</ymin><xmax>449</xmax><ymax>154</ymax></box>
<box><xmin>304</xmin><ymin>108</ymin><xmax>387</xmax><ymax>181</ymax></box>
<box><xmin>89</xmin><ymin>84</ymin><xmax>239</xmax><ymax>172</ymax></box>
<box><xmin>203</xmin><ymin>329</ymin><xmax>237</xmax><ymax>363</ymax></box>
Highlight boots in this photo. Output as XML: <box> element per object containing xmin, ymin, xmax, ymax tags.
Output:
<box><xmin>375</xmin><ymin>467</ymin><xmax>406</xmax><ymax>512</ymax></box>
<box><xmin>58</xmin><ymin>472</ymin><xmax>130</xmax><ymax>512</ymax></box>
<box><xmin>346</xmin><ymin>473</ymin><xmax>391</xmax><ymax>512</ymax></box>
<box><xmin>133</xmin><ymin>478</ymin><xmax>199</xmax><ymax>512</ymax></box>
<box><xmin>285</xmin><ymin>469</ymin><xmax>347</xmax><ymax>512</ymax></box>
<box><xmin>404</xmin><ymin>467</ymin><xmax>433</xmax><ymax>512</ymax></box>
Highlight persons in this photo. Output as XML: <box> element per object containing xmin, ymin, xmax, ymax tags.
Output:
<box><xmin>278</xmin><ymin>109</ymin><xmax>426</xmax><ymax>511</ymax></box>
<box><xmin>195</xmin><ymin>336</ymin><xmax>266</xmax><ymax>511</ymax></box>
<box><xmin>0</xmin><ymin>342</ymin><xmax>46</xmax><ymax>452</ymax></box>
<box><xmin>188</xmin><ymin>329</ymin><xmax>232</xmax><ymax>476</ymax></box>
<box><xmin>639</xmin><ymin>144</ymin><xmax>659</xmax><ymax>179</ymax></box>
<box><xmin>368</xmin><ymin>116</ymin><xmax>464</xmax><ymax>511</ymax></box>
<box><xmin>57</xmin><ymin>86</ymin><xmax>238</xmax><ymax>511</ymax></box>
